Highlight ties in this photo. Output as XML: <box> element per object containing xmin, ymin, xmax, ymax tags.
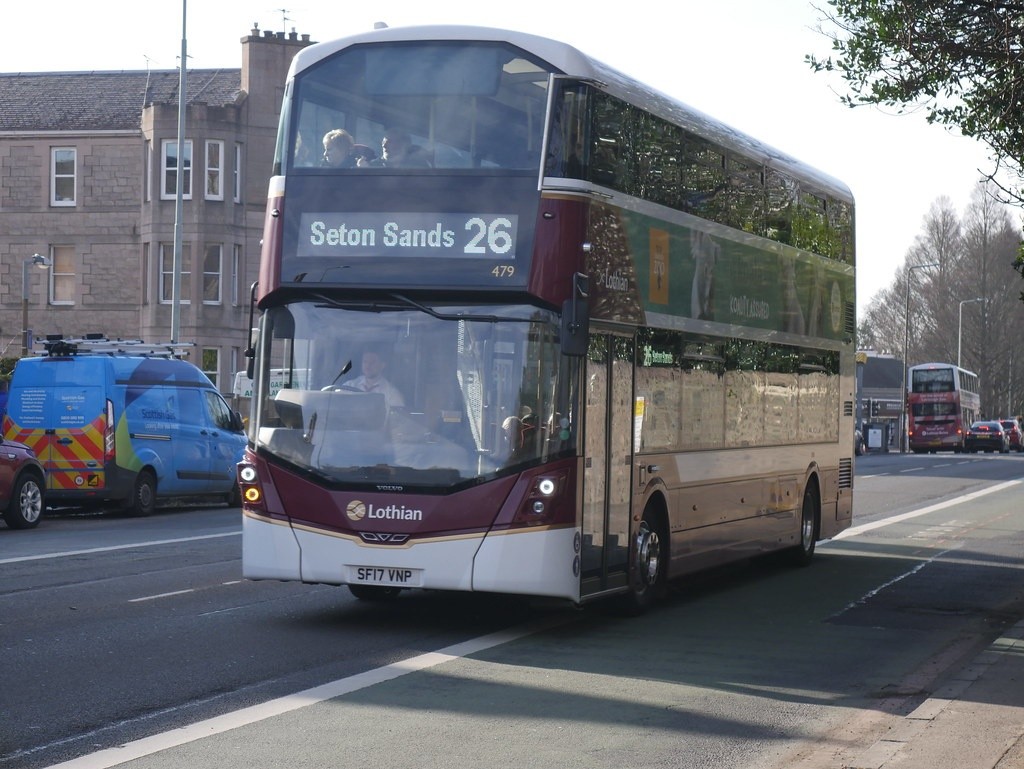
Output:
<box><xmin>364</xmin><ymin>384</ymin><xmax>379</xmax><ymax>392</ymax></box>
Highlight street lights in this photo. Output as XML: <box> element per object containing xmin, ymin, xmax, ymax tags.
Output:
<box><xmin>19</xmin><ymin>253</ymin><xmax>52</xmax><ymax>360</ymax></box>
<box><xmin>957</xmin><ymin>297</ymin><xmax>991</xmax><ymax>367</ymax></box>
<box><xmin>901</xmin><ymin>262</ymin><xmax>942</xmax><ymax>453</ymax></box>
<box><xmin>316</xmin><ymin>359</ymin><xmax>353</xmax><ymax>469</ymax></box>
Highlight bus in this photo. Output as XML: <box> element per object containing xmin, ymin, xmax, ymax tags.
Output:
<box><xmin>234</xmin><ymin>20</ymin><xmax>858</xmax><ymax>616</ymax></box>
<box><xmin>905</xmin><ymin>361</ymin><xmax>982</xmax><ymax>455</ymax></box>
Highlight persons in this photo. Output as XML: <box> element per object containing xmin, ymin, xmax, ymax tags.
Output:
<box><xmin>322</xmin><ymin>127</ymin><xmax>432</xmax><ymax>171</ymax></box>
<box><xmin>338</xmin><ymin>344</ymin><xmax>403</xmax><ymax>410</ymax></box>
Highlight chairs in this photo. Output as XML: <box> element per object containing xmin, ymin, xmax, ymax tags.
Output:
<box><xmin>352</xmin><ymin>144</ymin><xmax>437</xmax><ymax>169</ymax></box>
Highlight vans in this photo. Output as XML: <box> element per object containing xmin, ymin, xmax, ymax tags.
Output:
<box><xmin>0</xmin><ymin>332</ymin><xmax>249</xmax><ymax>518</ymax></box>
<box><xmin>231</xmin><ymin>365</ymin><xmax>311</xmax><ymax>401</ymax></box>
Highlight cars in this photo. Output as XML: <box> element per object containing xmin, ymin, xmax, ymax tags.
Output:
<box><xmin>965</xmin><ymin>420</ymin><xmax>1010</xmax><ymax>454</ymax></box>
<box><xmin>991</xmin><ymin>416</ymin><xmax>1024</xmax><ymax>452</ymax></box>
<box><xmin>0</xmin><ymin>433</ymin><xmax>48</xmax><ymax>529</ymax></box>
<box><xmin>855</xmin><ymin>428</ymin><xmax>867</xmax><ymax>456</ymax></box>
